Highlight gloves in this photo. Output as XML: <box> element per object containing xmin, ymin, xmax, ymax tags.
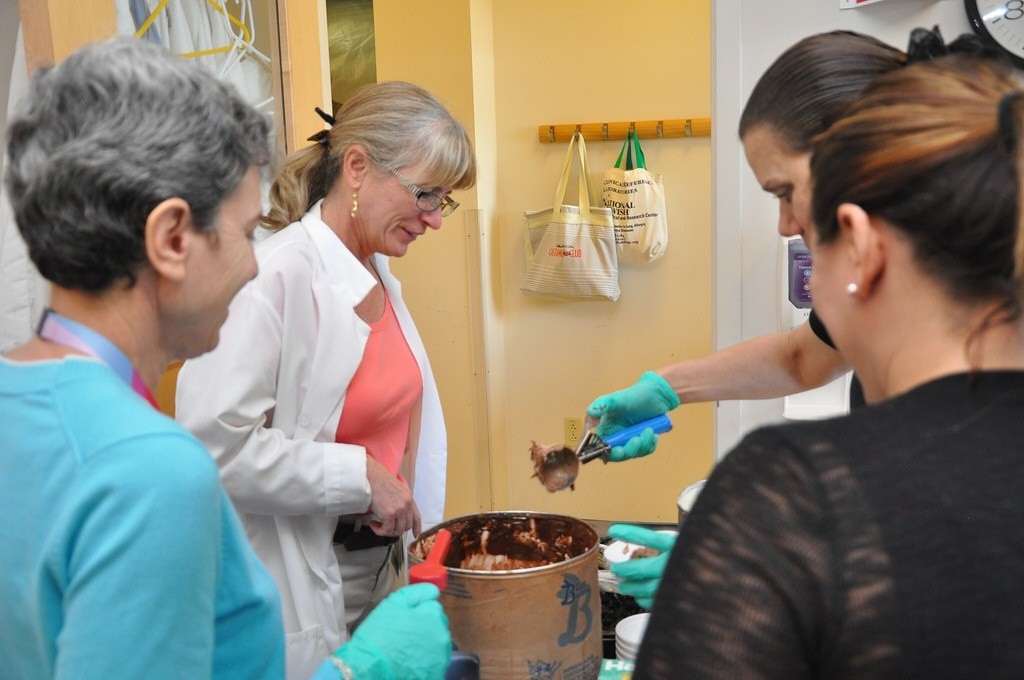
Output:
<box><xmin>609</xmin><ymin>522</ymin><xmax>679</xmax><ymax>609</ymax></box>
<box><xmin>332</xmin><ymin>582</ymin><xmax>451</xmax><ymax>680</ymax></box>
<box><xmin>586</xmin><ymin>371</ymin><xmax>679</xmax><ymax>463</ymax></box>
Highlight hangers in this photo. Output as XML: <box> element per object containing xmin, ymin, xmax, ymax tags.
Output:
<box><xmin>132</xmin><ymin>0</ymin><xmax>250</xmax><ymax>59</ymax></box>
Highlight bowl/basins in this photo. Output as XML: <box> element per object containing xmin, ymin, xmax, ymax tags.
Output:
<box><xmin>604</xmin><ymin>530</ymin><xmax>678</xmax><ymax>565</ymax></box>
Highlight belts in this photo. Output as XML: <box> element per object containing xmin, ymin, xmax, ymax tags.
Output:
<box><xmin>335</xmin><ymin>520</ymin><xmax>405</xmax><ymax>555</ymax></box>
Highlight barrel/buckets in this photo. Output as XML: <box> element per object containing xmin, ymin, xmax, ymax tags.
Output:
<box><xmin>406</xmin><ymin>508</ymin><xmax>602</xmax><ymax>680</ymax></box>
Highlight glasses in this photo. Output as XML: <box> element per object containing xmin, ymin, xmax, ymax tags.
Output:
<box><xmin>368</xmin><ymin>152</ymin><xmax>460</xmax><ymax>218</ymax></box>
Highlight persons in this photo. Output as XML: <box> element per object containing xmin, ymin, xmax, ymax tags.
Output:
<box><xmin>0</xmin><ymin>34</ymin><xmax>454</xmax><ymax>680</ymax></box>
<box><xmin>586</xmin><ymin>24</ymin><xmax>975</xmax><ymax>609</ymax></box>
<box><xmin>174</xmin><ymin>80</ymin><xmax>476</xmax><ymax>680</ymax></box>
<box><xmin>630</xmin><ymin>41</ymin><xmax>1024</xmax><ymax>680</ymax></box>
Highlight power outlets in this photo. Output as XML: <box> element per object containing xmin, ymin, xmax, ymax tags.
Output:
<box><xmin>563</xmin><ymin>419</ymin><xmax>582</xmax><ymax>447</ymax></box>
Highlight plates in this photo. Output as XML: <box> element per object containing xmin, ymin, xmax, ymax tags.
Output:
<box><xmin>615</xmin><ymin>612</ymin><xmax>651</xmax><ymax>661</ymax></box>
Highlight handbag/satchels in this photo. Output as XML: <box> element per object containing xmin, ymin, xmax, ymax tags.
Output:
<box><xmin>601</xmin><ymin>130</ymin><xmax>670</xmax><ymax>264</ymax></box>
<box><xmin>519</xmin><ymin>132</ymin><xmax>619</xmax><ymax>301</ymax></box>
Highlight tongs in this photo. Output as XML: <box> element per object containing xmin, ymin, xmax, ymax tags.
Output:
<box><xmin>537</xmin><ymin>414</ymin><xmax>673</xmax><ymax>491</ymax></box>
